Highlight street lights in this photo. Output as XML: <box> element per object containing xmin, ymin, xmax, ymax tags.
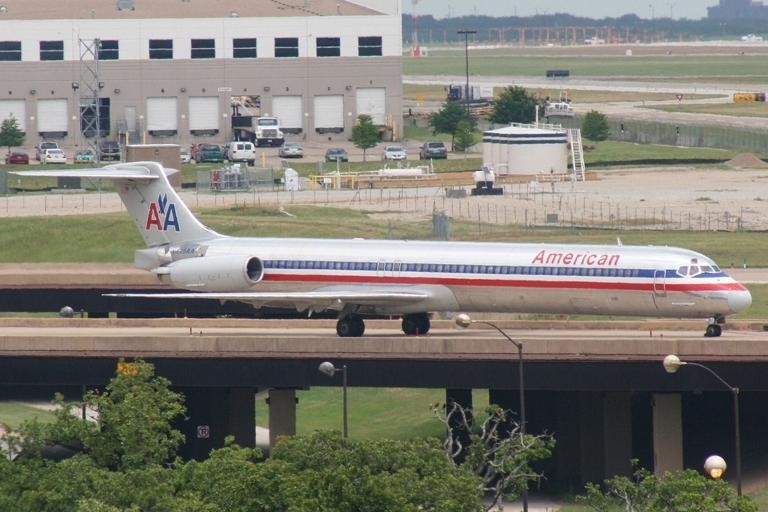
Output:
<box><xmin>319</xmin><ymin>361</ymin><xmax>347</xmax><ymax>460</ymax></box>
<box><xmin>223</xmin><ymin>113</ymin><xmax>229</xmax><ymax>142</ymax></box>
<box><xmin>56</xmin><ymin>306</ymin><xmax>85</xmax><ymax>423</ymax></box>
<box><xmin>348</xmin><ymin>111</ymin><xmax>352</xmax><ymax>140</ymax></box>
<box><xmin>457</xmin><ymin>29</ymin><xmax>476</xmax><ymax>119</ymax></box>
<box><xmin>264</xmin><ymin>113</ymin><xmax>269</xmax><ymax>116</ymax></box>
<box><xmin>139</xmin><ymin>115</ymin><xmax>144</xmax><ymax>144</ymax></box>
<box><xmin>30</xmin><ymin>116</ymin><xmax>34</xmax><ymax>151</ymax></box>
<box><xmin>304</xmin><ymin>113</ymin><xmax>309</xmax><ymax>143</ymax></box>
<box><xmin>662</xmin><ymin>356</ymin><xmax>742</xmax><ymax>498</ymax></box>
<box><xmin>71</xmin><ymin>115</ymin><xmax>77</xmax><ymax>146</ymax></box>
<box><xmin>454</xmin><ymin>313</ymin><xmax>529</xmax><ymax>512</ymax></box>
<box><xmin>181</xmin><ymin>115</ymin><xmax>187</xmax><ymax>144</ymax></box>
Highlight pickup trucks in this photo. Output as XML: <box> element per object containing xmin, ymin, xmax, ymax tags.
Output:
<box><xmin>191</xmin><ymin>142</ymin><xmax>225</xmax><ymax>164</ymax></box>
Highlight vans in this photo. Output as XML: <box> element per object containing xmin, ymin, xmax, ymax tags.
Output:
<box><xmin>229</xmin><ymin>142</ymin><xmax>256</xmax><ymax>166</ymax></box>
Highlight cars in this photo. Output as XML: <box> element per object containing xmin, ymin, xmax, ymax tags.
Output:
<box><xmin>34</xmin><ymin>141</ymin><xmax>67</xmax><ymax>164</ymax></box>
<box><xmin>181</xmin><ymin>148</ymin><xmax>190</xmax><ymax>164</ymax></box>
<box><xmin>5</xmin><ymin>150</ymin><xmax>29</xmax><ymax>164</ymax></box>
<box><xmin>278</xmin><ymin>142</ymin><xmax>303</xmax><ymax>158</ymax></box>
<box><xmin>74</xmin><ymin>150</ymin><xmax>97</xmax><ymax>163</ymax></box>
<box><xmin>325</xmin><ymin>148</ymin><xmax>347</xmax><ymax>162</ymax></box>
<box><xmin>382</xmin><ymin>146</ymin><xmax>407</xmax><ymax>161</ymax></box>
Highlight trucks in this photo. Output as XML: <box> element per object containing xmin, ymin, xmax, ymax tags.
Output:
<box><xmin>232</xmin><ymin>113</ymin><xmax>284</xmax><ymax>147</ymax></box>
<box><xmin>733</xmin><ymin>92</ymin><xmax>765</xmax><ymax>103</ymax></box>
<box><xmin>444</xmin><ymin>84</ymin><xmax>494</xmax><ymax>102</ymax></box>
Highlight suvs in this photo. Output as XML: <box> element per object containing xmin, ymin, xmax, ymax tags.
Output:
<box><xmin>419</xmin><ymin>141</ymin><xmax>447</xmax><ymax>159</ymax></box>
<box><xmin>99</xmin><ymin>140</ymin><xmax>120</xmax><ymax>160</ymax></box>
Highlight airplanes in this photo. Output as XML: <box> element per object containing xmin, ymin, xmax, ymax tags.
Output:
<box><xmin>8</xmin><ymin>161</ymin><xmax>752</xmax><ymax>337</ymax></box>
